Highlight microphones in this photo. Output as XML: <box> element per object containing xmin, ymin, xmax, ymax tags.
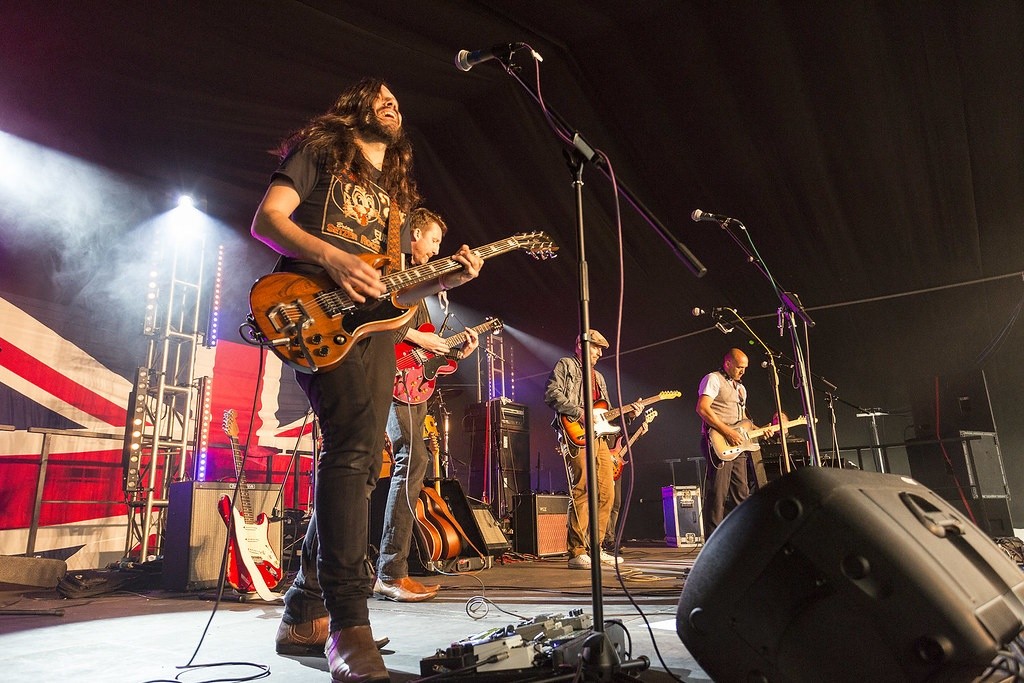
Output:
<box><xmin>761</xmin><ymin>361</ymin><xmax>784</xmax><ymax>368</ymax></box>
<box><xmin>455</xmin><ymin>42</ymin><xmax>526</xmax><ymax>71</ymax></box>
<box><xmin>692</xmin><ymin>306</ymin><xmax>728</xmax><ymax>315</ymax></box>
<box><xmin>692</xmin><ymin>210</ymin><xmax>742</xmax><ymax>225</ymax></box>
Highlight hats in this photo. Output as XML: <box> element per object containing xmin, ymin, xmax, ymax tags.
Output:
<box><xmin>576</xmin><ymin>329</ymin><xmax>610</xmax><ymax>349</ymax></box>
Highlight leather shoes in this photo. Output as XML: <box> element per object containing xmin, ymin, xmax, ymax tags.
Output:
<box><xmin>373</xmin><ymin>575</ymin><xmax>438</xmax><ymax>603</ymax></box>
<box><xmin>394</xmin><ymin>578</ymin><xmax>441</xmax><ymax>594</ymax></box>
<box><xmin>275</xmin><ymin>617</ymin><xmax>390</xmax><ymax>657</ymax></box>
<box><xmin>324</xmin><ymin>624</ymin><xmax>392</xmax><ymax>683</ymax></box>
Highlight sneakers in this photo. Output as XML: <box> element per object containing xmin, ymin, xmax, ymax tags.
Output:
<box><xmin>588</xmin><ymin>550</ymin><xmax>624</xmax><ymax>566</ymax></box>
<box><xmin>568</xmin><ymin>555</ymin><xmax>595</xmax><ymax>570</ymax></box>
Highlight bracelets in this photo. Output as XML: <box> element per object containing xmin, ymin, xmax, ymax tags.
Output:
<box><xmin>439</xmin><ymin>275</ymin><xmax>453</xmax><ymax>293</ymax></box>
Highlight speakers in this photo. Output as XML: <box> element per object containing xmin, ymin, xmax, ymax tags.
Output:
<box><xmin>163</xmin><ymin>481</ymin><xmax>284</xmax><ymax>591</ymax></box>
<box><xmin>470</xmin><ymin>428</ymin><xmax>531</xmax><ymax>472</ymax></box>
<box><xmin>468</xmin><ymin>473</ymin><xmax>531</xmax><ymax>527</ymax></box>
<box><xmin>675</xmin><ymin>466</ymin><xmax>1024</xmax><ymax>683</ymax></box>
<box><xmin>470</xmin><ymin>505</ymin><xmax>512</xmax><ymax>561</ymax></box>
<box><xmin>513</xmin><ymin>493</ymin><xmax>571</xmax><ymax>558</ymax></box>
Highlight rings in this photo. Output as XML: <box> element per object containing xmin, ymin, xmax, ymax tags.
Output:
<box><xmin>467</xmin><ymin>340</ymin><xmax>471</xmax><ymax>344</ymax></box>
<box><xmin>471</xmin><ymin>335</ymin><xmax>478</xmax><ymax>342</ymax></box>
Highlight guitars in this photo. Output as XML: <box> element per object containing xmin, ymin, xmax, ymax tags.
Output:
<box><xmin>247</xmin><ymin>231</ymin><xmax>560</xmax><ymax>374</ymax></box>
<box><xmin>392</xmin><ymin>315</ymin><xmax>505</xmax><ymax>405</ymax></box>
<box><xmin>709</xmin><ymin>415</ymin><xmax>819</xmax><ymax>462</ymax></box>
<box><xmin>608</xmin><ymin>407</ymin><xmax>659</xmax><ymax>481</ymax></box>
<box><xmin>560</xmin><ymin>390</ymin><xmax>683</xmax><ymax>446</ymax></box>
<box><xmin>383</xmin><ymin>414</ymin><xmax>463</xmax><ymax>563</ymax></box>
<box><xmin>217</xmin><ymin>408</ymin><xmax>283</xmax><ymax>594</ymax></box>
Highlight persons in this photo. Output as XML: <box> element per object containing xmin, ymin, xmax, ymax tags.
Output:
<box><xmin>544</xmin><ymin>329</ymin><xmax>648</xmax><ymax>570</ymax></box>
<box><xmin>373</xmin><ymin>207</ymin><xmax>480</xmax><ymax>603</ymax></box>
<box><xmin>761</xmin><ymin>409</ymin><xmax>804</xmax><ymax>446</ymax></box>
<box><xmin>251</xmin><ymin>77</ymin><xmax>484</xmax><ymax>683</ymax></box>
<box><xmin>696</xmin><ymin>348</ymin><xmax>774</xmax><ymax>545</ymax></box>
